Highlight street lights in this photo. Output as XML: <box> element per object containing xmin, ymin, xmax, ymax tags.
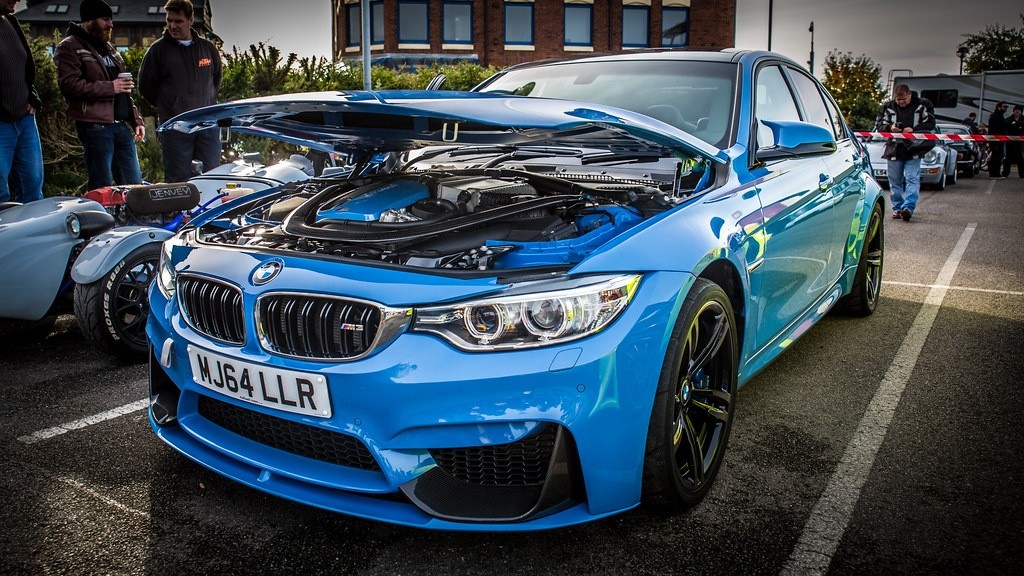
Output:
<box><xmin>956</xmin><ymin>44</ymin><xmax>970</xmax><ymax>74</ymax></box>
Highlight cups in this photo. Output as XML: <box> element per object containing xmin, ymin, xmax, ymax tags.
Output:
<box><xmin>118</xmin><ymin>72</ymin><xmax>132</xmax><ymax>93</ymax></box>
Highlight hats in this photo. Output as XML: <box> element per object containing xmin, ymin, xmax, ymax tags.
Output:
<box><xmin>80</xmin><ymin>0</ymin><xmax>112</xmax><ymax>23</ymax></box>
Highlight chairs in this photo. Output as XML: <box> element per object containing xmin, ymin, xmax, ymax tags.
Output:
<box><xmin>645</xmin><ymin>104</ymin><xmax>696</xmax><ymax>133</ymax></box>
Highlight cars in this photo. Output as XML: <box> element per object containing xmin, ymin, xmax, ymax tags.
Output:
<box><xmin>855</xmin><ymin>121</ymin><xmax>983</xmax><ymax>191</ymax></box>
<box><xmin>145</xmin><ymin>51</ymin><xmax>886</xmax><ymax>532</ymax></box>
<box><xmin>0</xmin><ymin>150</ymin><xmax>345</xmax><ymax>366</ymax></box>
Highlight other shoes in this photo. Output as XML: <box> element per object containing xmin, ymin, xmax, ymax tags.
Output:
<box><xmin>893</xmin><ymin>211</ymin><xmax>902</xmax><ymax>219</ymax></box>
<box><xmin>989</xmin><ymin>174</ymin><xmax>1006</xmax><ymax>179</ymax></box>
<box><xmin>902</xmin><ymin>208</ymin><xmax>911</xmax><ymax>222</ymax></box>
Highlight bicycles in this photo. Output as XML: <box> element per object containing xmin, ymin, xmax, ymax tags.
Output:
<box><xmin>978</xmin><ymin>139</ymin><xmax>1007</xmax><ymax>171</ymax></box>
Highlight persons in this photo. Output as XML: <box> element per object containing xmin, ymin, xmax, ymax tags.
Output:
<box><xmin>54</xmin><ymin>0</ymin><xmax>146</xmax><ymax>192</ymax></box>
<box><xmin>962</xmin><ymin>112</ymin><xmax>989</xmax><ymax>168</ymax></box>
<box><xmin>139</xmin><ymin>0</ymin><xmax>223</xmax><ymax>183</ymax></box>
<box><xmin>0</xmin><ymin>0</ymin><xmax>45</xmax><ymax>209</ymax></box>
<box><xmin>877</xmin><ymin>84</ymin><xmax>937</xmax><ymax>221</ymax></box>
<box><xmin>988</xmin><ymin>102</ymin><xmax>1024</xmax><ymax>179</ymax></box>
